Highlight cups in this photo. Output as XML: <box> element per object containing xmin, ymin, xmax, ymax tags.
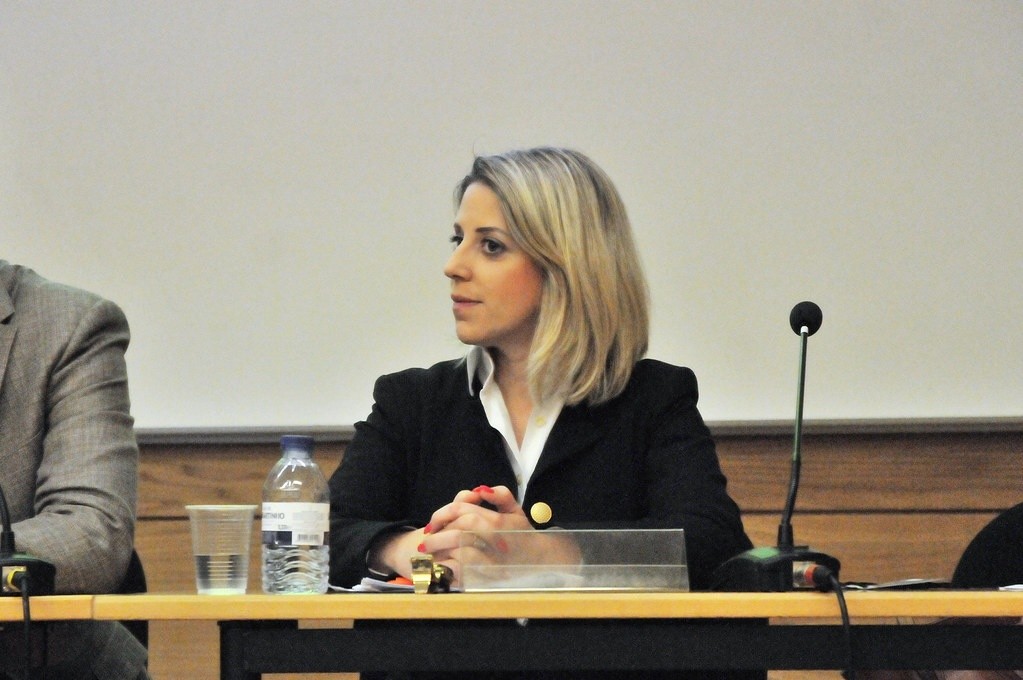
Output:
<box><xmin>185</xmin><ymin>504</ymin><xmax>258</xmax><ymax>594</ymax></box>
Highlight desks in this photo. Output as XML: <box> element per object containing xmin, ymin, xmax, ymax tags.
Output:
<box><xmin>0</xmin><ymin>587</ymin><xmax>1023</xmax><ymax>680</ymax></box>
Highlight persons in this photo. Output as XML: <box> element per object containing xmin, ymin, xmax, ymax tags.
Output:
<box><xmin>329</xmin><ymin>147</ymin><xmax>768</xmax><ymax>680</ymax></box>
<box><xmin>0</xmin><ymin>261</ymin><xmax>149</xmax><ymax>680</ymax></box>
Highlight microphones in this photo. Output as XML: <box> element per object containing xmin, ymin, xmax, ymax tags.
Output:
<box><xmin>708</xmin><ymin>301</ymin><xmax>841</xmax><ymax>595</ymax></box>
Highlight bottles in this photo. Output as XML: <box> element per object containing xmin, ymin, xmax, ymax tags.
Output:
<box><xmin>261</xmin><ymin>435</ymin><xmax>331</xmax><ymax>594</ymax></box>
